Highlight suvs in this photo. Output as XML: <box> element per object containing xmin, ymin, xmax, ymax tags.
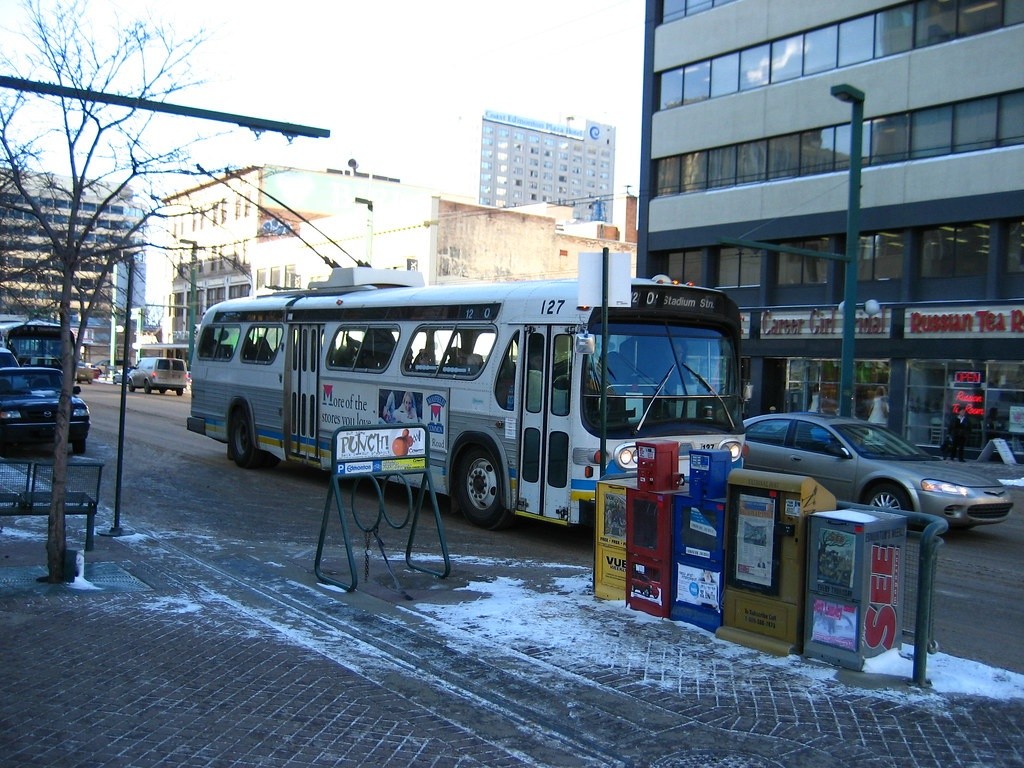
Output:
<box><xmin>127</xmin><ymin>357</ymin><xmax>188</xmax><ymax>396</ymax></box>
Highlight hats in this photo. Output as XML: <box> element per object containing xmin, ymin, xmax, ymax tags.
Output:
<box><xmin>671</xmin><ymin>342</ymin><xmax>683</xmax><ymax>352</ymax></box>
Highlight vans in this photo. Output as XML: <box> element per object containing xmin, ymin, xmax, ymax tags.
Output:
<box><xmin>95</xmin><ymin>359</ymin><xmax>132</xmax><ymax>376</ymax></box>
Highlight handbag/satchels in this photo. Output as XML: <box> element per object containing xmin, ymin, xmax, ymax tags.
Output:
<box><xmin>940</xmin><ymin>437</ymin><xmax>953</xmax><ymax>455</ymax></box>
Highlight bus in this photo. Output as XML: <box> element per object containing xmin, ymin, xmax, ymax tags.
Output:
<box><xmin>186</xmin><ymin>265</ymin><xmax>747</xmax><ymax>529</ymax></box>
<box><xmin>0</xmin><ymin>320</ymin><xmax>77</xmax><ymax>388</ymax></box>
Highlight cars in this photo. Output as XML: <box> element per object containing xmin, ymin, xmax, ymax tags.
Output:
<box><xmin>741</xmin><ymin>412</ymin><xmax>1013</xmax><ymax>534</ymax></box>
<box><xmin>0</xmin><ymin>347</ymin><xmax>30</xmax><ymax>393</ymax></box>
<box><xmin>111</xmin><ymin>366</ymin><xmax>136</xmax><ymax>386</ymax></box>
<box><xmin>0</xmin><ymin>367</ymin><xmax>91</xmax><ymax>454</ymax></box>
<box><xmin>75</xmin><ymin>361</ymin><xmax>100</xmax><ymax>384</ymax></box>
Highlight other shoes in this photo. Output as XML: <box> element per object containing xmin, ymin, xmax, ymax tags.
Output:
<box><xmin>951</xmin><ymin>455</ymin><xmax>954</xmax><ymax>460</ymax></box>
<box><xmin>959</xmin><ymin>459</ymin><xmax>965</xmax><ymax>463</ymax></box>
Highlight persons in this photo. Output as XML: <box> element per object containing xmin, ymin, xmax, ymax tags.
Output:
<box><xmin>946</xmin><ymin>406</ymin><xmax>972</xmax><ymax>463</ymax></box>
<box><xmin>379</xmin><ymin>391</ymin><xmax>419</xmax><ymax>423</ymax></box>
<box><xmin>651</xmin><ymin>340</ymin><xmax>696</xmax><ymax>397</ymax></box>
<box><xmin>808</xmin><ymin>383</ymin><xmax>823</xmax><ymax>413</ymax></box>
<box><xmin>699</xmin><ymin>570</ymin><xmax>718</xmax><ymax>608</ymax></box>
<box><xmin>867</xmin><ymin>386</ymin><xmax>889</xmax><ymax>428</ymax></box>
<box><xmin>985</xmin><ymin>406</ymin><xmax>1013</xmax><ymax>441</ymax></box>
<box><xmin>246</xmin><ymin>328</ymin><xmax>484</xmax><ymax>369</ymax></box>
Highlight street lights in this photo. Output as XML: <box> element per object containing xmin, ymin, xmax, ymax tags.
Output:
<box><xmin>97</xmin><ymin>249</ymin><xmax>135</xmax><ymax>538</ymax></box>
<box><xmin>829</xmin><ymin>84</ymin><xmax>869</xmax><ymax>418</ymax></box>
<box><xmin>179</xmin><ymin>239</ymin><xmax>198</xmax><ymax>371</ymax></box>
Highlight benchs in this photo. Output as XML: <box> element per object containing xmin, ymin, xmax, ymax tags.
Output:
<box><xmin>0</xmin><ymin>460</ymin><xmax>105</xmax><ymax>552</ymax></box>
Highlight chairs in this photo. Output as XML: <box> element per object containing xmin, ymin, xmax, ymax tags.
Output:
<box><xmin>33</xmin><ymin>379</ymin><xmax>50</xmax><ymax>388</ymax></box>
<box><xmin>466</xmin><ymin>354</ymin><xmax>484</xmax><ymax>365</ymax></box>
<box><xmin>242</xmin><ymin>337</ymin><xmax>274</xmax><ymax>361</ymax></box>
<box><xmin>0</xmin><ymin>379</ymin><xmax>12</xmax><ymax>392</ymax></box>
<box><xmin>409</xmin><ymin>349</ymin><xmax>437</xmax><ymax>373</ymax></box>
